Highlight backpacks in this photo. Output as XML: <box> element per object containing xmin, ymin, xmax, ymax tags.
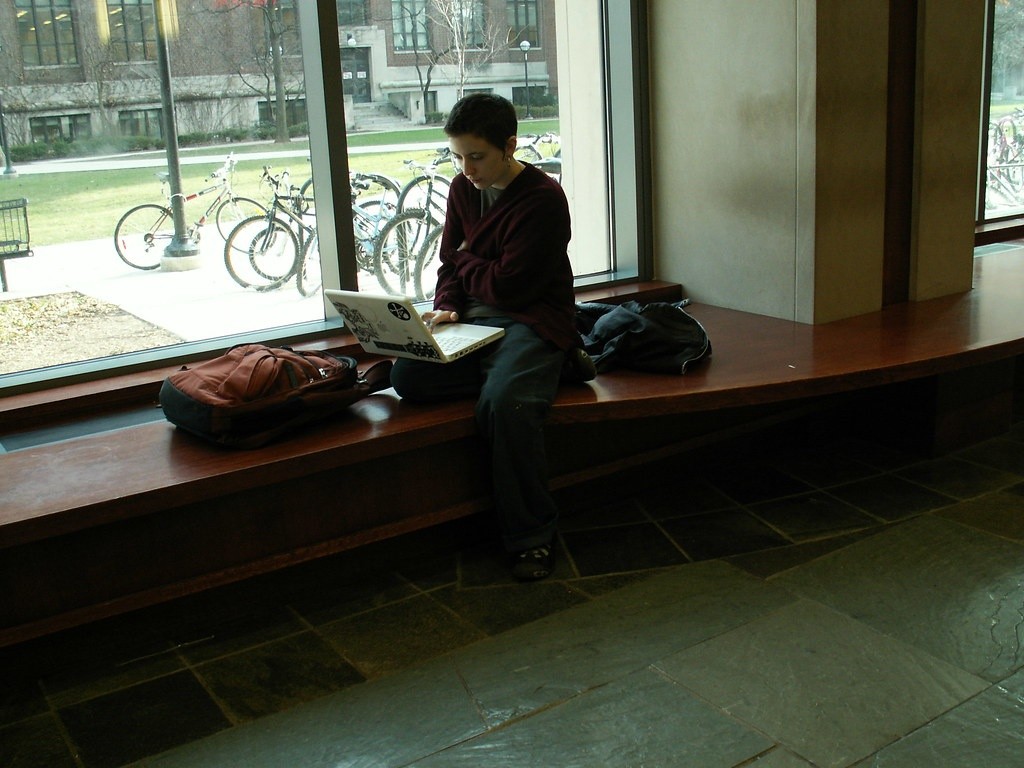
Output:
<box><xmin>159</xmin><ymin>343</ymin><xmax>393</xmax><ymax>450</ymax></box>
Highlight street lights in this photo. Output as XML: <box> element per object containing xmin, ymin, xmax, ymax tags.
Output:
<box><xmin>519</xmin><ymin>40</ymin><xmax>535</xmax><ymax>120</ymax></box>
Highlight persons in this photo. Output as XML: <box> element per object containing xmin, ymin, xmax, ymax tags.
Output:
<box><xmin>388</xmin><ymin>93</ymin><xmax>578</xmax><ymax>585</ymax></box>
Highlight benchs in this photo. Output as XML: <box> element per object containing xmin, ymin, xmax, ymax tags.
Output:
<box><xmin>0</xmin><ymin>246</ymin><xmax>1024</xmax><ymax>647</ymax></box>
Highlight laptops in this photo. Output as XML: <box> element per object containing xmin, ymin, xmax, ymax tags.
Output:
<box><xmin>324</xmin><ymin>290</ymin><xmax>506</xmax><ymax>364</ymax></box>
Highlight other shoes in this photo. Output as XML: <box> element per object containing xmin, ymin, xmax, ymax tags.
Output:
<box><xmin>516</xmin><ymin>528</ymin><xmax>556</xmax><ymax>580</ymax></box>
<box><xmin>573</xmin><ymin>348</ymin><xmax>598</xmax><ymax>382</ymax></box>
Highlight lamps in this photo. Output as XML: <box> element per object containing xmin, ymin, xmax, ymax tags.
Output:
<box><xmin>346</xmin><ymin>32</ymin><xmax>357</xmax><ymax>48</ymax></box>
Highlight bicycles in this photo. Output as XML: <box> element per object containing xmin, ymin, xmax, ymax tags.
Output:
<box><xmin>984</xmin><ymin>108</ymin><xmax>1024</xmax><ymax>207</ymax></box>
<box><xmin>113</xmin><ymin>150</ymin><xmax>278</xmax><ymax>272</ymax></box>
<box><xmin>224</xmin><ymin>147</ymin><xmax>465</xmax><ymax>304</ymax></box>
<box><xmin>513</xmin><ymin>131</ymin><xmax>562</xmax><ymax>183</ymax></box>
